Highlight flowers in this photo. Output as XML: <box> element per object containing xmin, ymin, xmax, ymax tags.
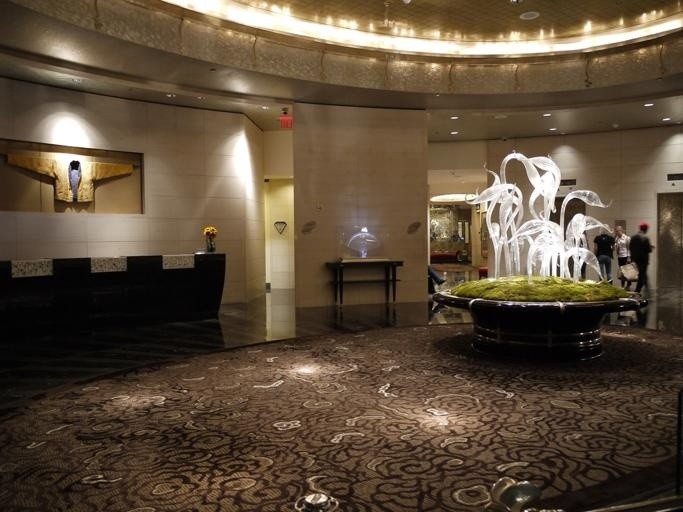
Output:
<box><xmin>203</xmin><ymin>226</ymin><xmax>218</xmax><ymax>249</ymax></box>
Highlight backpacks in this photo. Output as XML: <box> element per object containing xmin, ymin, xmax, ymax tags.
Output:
<box><xmin>621</xmin><ymin>261</ymin><xmax>639</xmax><ymax>281</ymax></box>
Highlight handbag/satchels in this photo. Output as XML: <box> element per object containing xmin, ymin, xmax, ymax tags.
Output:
<box><xmin>617</xmin><ymin>260</ymin><xmax>641</xmax><ymax>281</ymax></box>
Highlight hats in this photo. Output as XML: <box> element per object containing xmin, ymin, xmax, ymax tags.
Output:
<box><xmin>639</xmin><ymin>219</ymin><xmax>649</xmax><ymax>233</ymax></box>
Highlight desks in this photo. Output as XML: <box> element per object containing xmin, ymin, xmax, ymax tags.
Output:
<box><xmin>325</xmin><ymin>260</ymin><xmax>404</xmax><ymax>307</ymax></box>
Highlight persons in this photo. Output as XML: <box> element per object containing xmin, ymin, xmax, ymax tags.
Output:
<box><xmin>612</xmin><ymin>224</ymin><xmax>631</xmax><ymax>292</ymax></box>
<box><xmin>626</xmin><ymin>221</ymin><xmax>654</xmax><ymax>297</ymax></box>
<box><xmin>427</xmin><ymin>265</ymin><xmax>446</xmax><ymax>293</ymax></box>
<box><xmin>593</xmin><ymin>223</ymin><xmax>615</xmax><ymax>286</ymax></box>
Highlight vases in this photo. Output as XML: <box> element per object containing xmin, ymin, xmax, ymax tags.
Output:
<box><xmin>207</xmin><ymin>238</ymin><xmax>216</xmax><ymax>252</ymax></box>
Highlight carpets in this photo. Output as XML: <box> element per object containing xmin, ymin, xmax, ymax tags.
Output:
<box><xmin>1</xmin><ymin>322</ymin><xmax>683</xmax><ymax>512</ymax></box>
